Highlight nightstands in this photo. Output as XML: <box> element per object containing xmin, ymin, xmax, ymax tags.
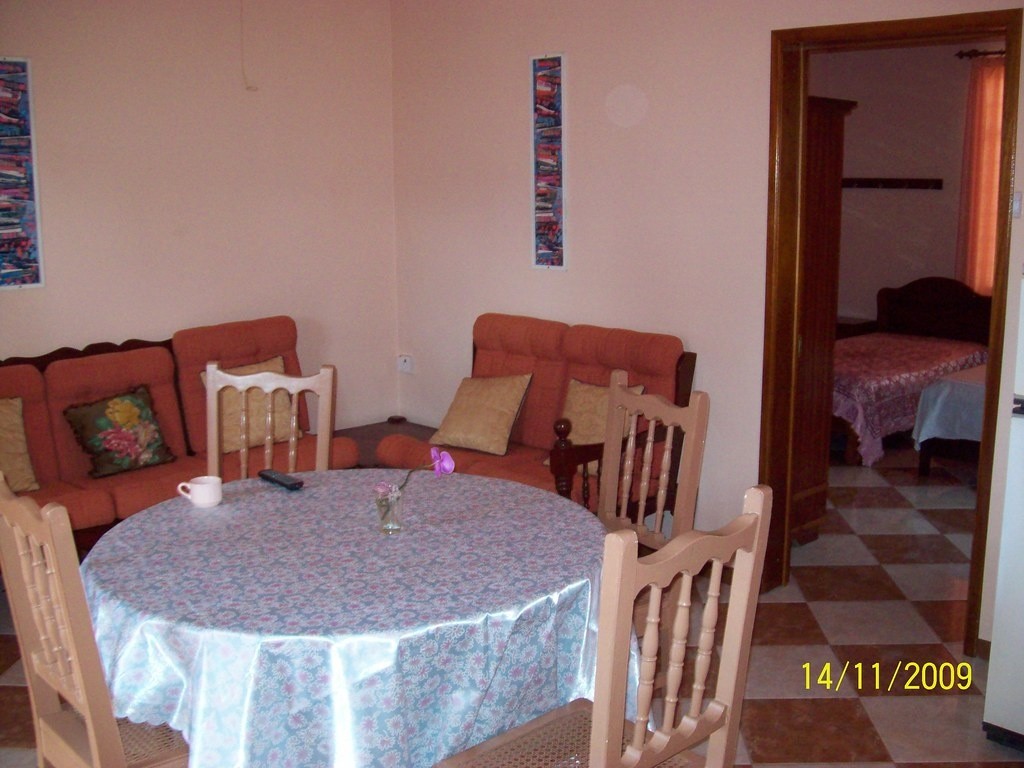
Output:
<box><xmin>838</xmin><ymin>315</ymin><xmax>877</xmax><ymax>336</ymax></box>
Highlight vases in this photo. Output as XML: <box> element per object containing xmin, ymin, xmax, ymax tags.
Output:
<box><xmin>375</xmin><ymin>490</ymin><xmax>402</xmax><ymax>534</ymax></box>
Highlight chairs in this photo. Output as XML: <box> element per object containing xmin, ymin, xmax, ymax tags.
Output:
<box><xmin>597</xmin><ymin>370</ymin><xmax>711</xmax><ymax>728</ymax></box>
<box><xmin>433</xmin><ymin>485</ymin><xmax>774</xmax><ymax>768</ymax></box>
<box><xmin>205</xmin><ymin>360</ymin><xmax>337</xmax><ymax>482</ymax></box>
<box><xmin>0</xmin><ymin>469</ymin><xmax>189</xmax><ymax>768</ymax></box>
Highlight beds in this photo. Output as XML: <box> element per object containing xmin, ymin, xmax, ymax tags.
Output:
<box><xmin>832</xmin><ymin>277</ymin><xmax>992</xmax><ymax>466</ymax></box>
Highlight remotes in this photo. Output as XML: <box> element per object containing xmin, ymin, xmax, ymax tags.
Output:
<box><xmin>257</xmin><ymin>469</ymin><xmax>304</xmax><ymax>491</ymax></box>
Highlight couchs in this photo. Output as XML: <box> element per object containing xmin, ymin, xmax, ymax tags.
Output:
<box><xmin>375</xmin><ymin>314</ymin><xmax>697</xmax><ymax>524</ymax></box>
<box><xmin>0</xmin><ymin>315</ymin><xmax>364</xmax><ymax>551</ymax></box>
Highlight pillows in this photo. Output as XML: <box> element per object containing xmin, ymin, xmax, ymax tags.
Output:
<box><xmin>543</xmin><ymin>378</ymin><xmax>644</xmax><ymax>477</ymax></box>
<box><xmin>200</xmin><ymin>356</ymin><xmax>302</xmax><ymax>455</ymax></box>
<box><xmin>428</xmin><ymin>373</ymin><xmax>532</xmax><ymax>456</ymax></box>
<box><xmin>0</xmin><ymin>396</ymin><xmax>39</xmax><ymax>492</ymax></box>
<box><xmin>63</xmin><ymin>383</ymin><xmax>179</xmax><ymax>478</ymax></box>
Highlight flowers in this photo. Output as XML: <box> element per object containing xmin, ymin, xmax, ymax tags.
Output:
<box><xmin>373</xmin><ymin>445</ymin><xmax>456</xmax><ymax>519</ymax></box>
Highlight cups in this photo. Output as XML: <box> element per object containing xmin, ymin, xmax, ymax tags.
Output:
<box><xmin>177</xmin><ymin>475</ymin><xmax>222</xmax><ymax>508</ymax></box>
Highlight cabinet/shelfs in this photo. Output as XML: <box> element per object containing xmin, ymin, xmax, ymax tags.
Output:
<box><xmin>786</xmin><ymin>94</ymin><xmax>860</xmax><ymax>546</ymax></box>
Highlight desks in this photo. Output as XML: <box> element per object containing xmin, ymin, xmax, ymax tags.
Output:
<box><xmin>332</xmin><ymin>415</ymin><xmax>439</xmax><ymax>468</ymax></box>
<box><xmin>910</xmin><ymin>363</ymin><xmax>987</xmax><ymax>478</ymax></box>
